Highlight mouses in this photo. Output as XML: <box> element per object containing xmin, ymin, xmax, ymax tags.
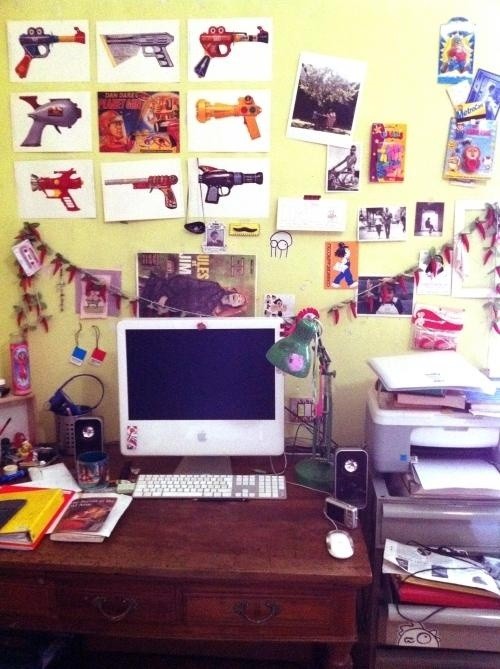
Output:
<box><xmin>326</xmin><ymin>530</ymin><xmax>355</xmax><ymax>560</ymax></box>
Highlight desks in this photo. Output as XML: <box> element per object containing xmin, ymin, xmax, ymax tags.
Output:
<box><xmin>2</xmin><ymin>444</ymin><xmax>373</xmax><ymax>668</ymax></box>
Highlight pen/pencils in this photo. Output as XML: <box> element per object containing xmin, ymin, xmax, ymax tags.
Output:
<box><xmin>0</xmin><ymin>417</ymin><xmax>11</xmax><ymax>436</ymax></box>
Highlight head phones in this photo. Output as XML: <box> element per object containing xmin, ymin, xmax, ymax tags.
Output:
<box><xmin>46</xmin><ymin>374</ymin><xmax>104</xmax><ymax>415</ymax></box>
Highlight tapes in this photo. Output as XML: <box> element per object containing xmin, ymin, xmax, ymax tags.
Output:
<box><xmin>2</xmin><ymin>464</ymin><xmax>18</xmax><ymax>476</ymax></box>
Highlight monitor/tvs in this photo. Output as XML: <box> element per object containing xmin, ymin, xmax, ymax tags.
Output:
<box><xmin>116</xmin><ymin>316</ymin><xmax>284</xmax><ymax>475</ymax></box>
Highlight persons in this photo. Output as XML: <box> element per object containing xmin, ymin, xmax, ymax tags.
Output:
<box><xmin>333</xmin><ymin>242</ymin><xmax>359</xmax><ymax>290</ymax></box>
<box><xmin>383</xmin><ymin>208</ymin><xmax>393</xmax><ymax>240</ymax></box>
<box><xmin>400</xmin><ymin>211</ymin><xmax>406</xmax><ymax>234</ymax></box>
<box><xmin>425</xmin><ymin>217</ymin><xmax>437</xmax><ymax>235</ymax></box>
<box><xmin>328</xmin><ymin>144</ymin><xmax>359</xmax><ymax>194</ymax></box>
<box><xmin>373</xmin><ymin>210</ymin><xmax>385</xmax><ymax>239</ymax></box>
<box><xmin>99</xmin><ymin>108</ymin><xmax>138</xmax><ymax>155</ymax></box>
<box><xmin>372</xmin><ymin>281</ymin><xmax>403</xmax><ymax>314</ymax></box>
<box><xmin>138</xmin><ymin>269</ymin><xmax>251</xmax><ymax>318</ymax></box>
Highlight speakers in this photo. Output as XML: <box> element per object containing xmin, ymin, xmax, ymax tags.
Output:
<box><xmin>335</xmin><ymin>447</ymin><xmax>369</xmax><ymax>511</ymax></box>
<box><xmin>74</xmin><ymin>415</ymin><xmax>104</xmax><ymax>464</ymax></box>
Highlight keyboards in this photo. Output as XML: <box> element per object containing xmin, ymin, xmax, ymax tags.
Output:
<box><xmin>132</xmin><ymin>474</ymin><xmax>287</xmax><ymax>501</ymax></box>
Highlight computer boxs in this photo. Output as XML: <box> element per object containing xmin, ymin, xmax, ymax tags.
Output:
<box><xmin>0</xmin><ymin>629</ymin><xmax>84</xmax><ymax>669</ymax></box>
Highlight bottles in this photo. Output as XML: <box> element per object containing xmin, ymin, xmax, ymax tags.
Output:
<box><xmin>7</xmin><ymin>332</ymin><xmax>31</xmax><ymax>395</ymax></box>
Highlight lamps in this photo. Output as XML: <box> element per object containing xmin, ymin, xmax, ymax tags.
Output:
<box><xmin>265</xmin><ymin>318</ymin><xmax>337</xmax><ymax>493</ymax></box>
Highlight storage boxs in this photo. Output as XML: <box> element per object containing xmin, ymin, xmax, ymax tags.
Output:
<box><xmin>363</xmin><ymin>389</ymin><xmax>500</xmax><ymax>474</ymax></box>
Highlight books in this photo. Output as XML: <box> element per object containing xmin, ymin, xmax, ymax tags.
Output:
<box><xmin>387</xmin><ymin>572</ymin><xmax>500</xmax><ymax>612</ymax></box>
<box><xmin>364</xmin><ymin>345</ymin><xmax>500</xmax><ymax>420</ymax></box>
<box><xmin>0</xmin><ymin>484</ymin><xmax>133</xmax><ymax>552</ymax></box>
<box><xmin>378</xmin><ymin>536</ymin><xmax>500</xmax><ymax>600</ymax></box>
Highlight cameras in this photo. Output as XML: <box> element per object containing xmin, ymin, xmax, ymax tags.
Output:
<box><xmin>325</xmin><ymin>496</ymin><xmax>358</xmax><ymax>529</ymax></box>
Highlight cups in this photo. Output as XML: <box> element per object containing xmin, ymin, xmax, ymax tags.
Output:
<box><xmin>76</xmin><ymin>451</ymin><xmax>109</xmax><ymax>492</ymax></box>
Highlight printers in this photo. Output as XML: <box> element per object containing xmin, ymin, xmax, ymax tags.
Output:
<box><xmin>363</xmin><ymin>382</ymin><xmax>500</xmax><ymax>561</ymax></box>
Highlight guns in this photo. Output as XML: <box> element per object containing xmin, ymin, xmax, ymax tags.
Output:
<box><xmin>15</xmin><ymin>27</ymin><xmax>85</xmax><ymax>78</ymax></box>
<box><xmin>196</xmin><ymin>96</ymin><xmax>261</xmax><ymax>140</ymax></box>
<box><xmin>194</xmin><ymin>26</ymin><xmax>268</xmax><ymax>78</ymax></box>
<box><xmin>105</xmin><ymin>175</ymin><xmax>177</xmax><ymax>208</ymax></box>
<box><xmin>20</xmin><ymin>96</ymin><xmax>81</xmax><ymax>147</ymax></box>
<box><xmin>31</xmin><ymin>169</ymin><xmax>83</xmax><ymax>211</ymax></box>
<box><xmin>198</xmin><ymin>165</ymin><xmax>262</xmax><ymax>204</ymax></box>
<box><xmin>101</xmin><ymin>33</ymin><xmax>174</xmax><ymax>67</ymax></box>
